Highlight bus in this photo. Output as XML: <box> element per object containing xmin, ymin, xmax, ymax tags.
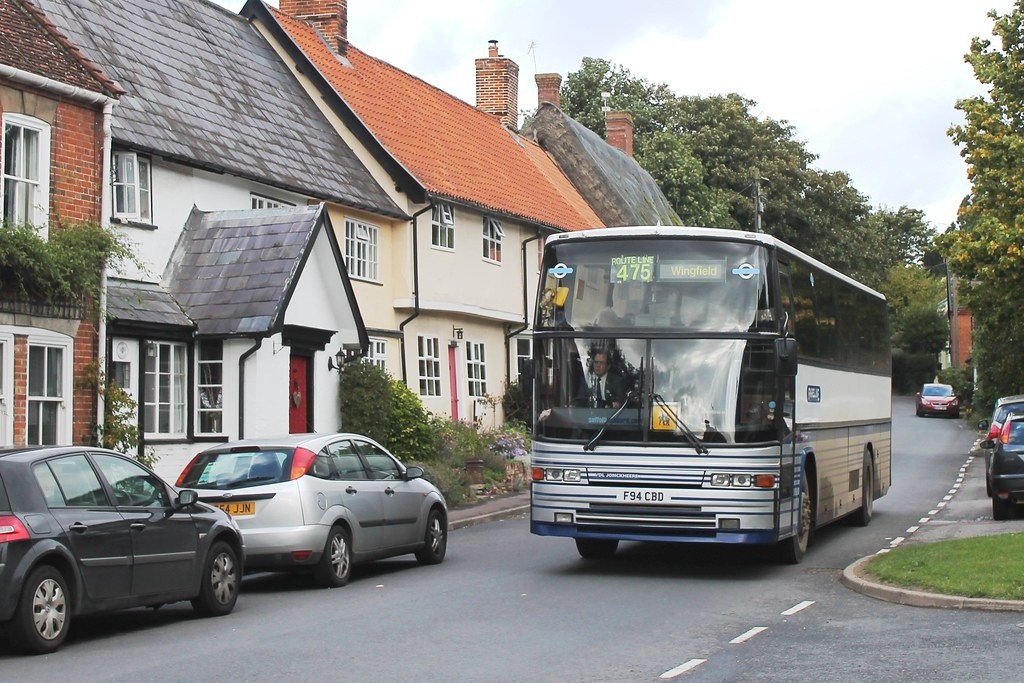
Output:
<box><xmin>504</xmin><ymin>220</ymin><xmax>893</xmax><ymax>564</ymax></box>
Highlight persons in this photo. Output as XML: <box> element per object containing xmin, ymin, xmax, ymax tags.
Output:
<box><xmin>571</xmin><ymin>351</ymin><xmax>626</xmax><ymax>409</ymax></box>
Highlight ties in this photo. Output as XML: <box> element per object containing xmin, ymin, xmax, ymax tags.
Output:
<box><xmin>596</xmin><ymin>377</ymin><xmax>601</xmax><ymax>402</ymax></box>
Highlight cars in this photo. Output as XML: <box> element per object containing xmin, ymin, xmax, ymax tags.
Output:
<box><xmin>171</xmin><ymin>432</ymin><xmax>449</xmax><ymax>587</ymax></box>
<box><xmin>980</xmin><ymin>408</ymin><xmax>1023</xmax><ymax>521</ymax></box>
<box><xmin>0</xmin><ymin>445</ymin><xmax>247</xmax><ymax>654</ymax></box>
<box><xmin>979</xmin><ymin>395</ymin><xmax>1024</xmax><ymax>469</ymax></box>
<box><xmin>915</xmin><ymin>383</ymin><xmax>960</xmax><ymax>418</ymax></box>
